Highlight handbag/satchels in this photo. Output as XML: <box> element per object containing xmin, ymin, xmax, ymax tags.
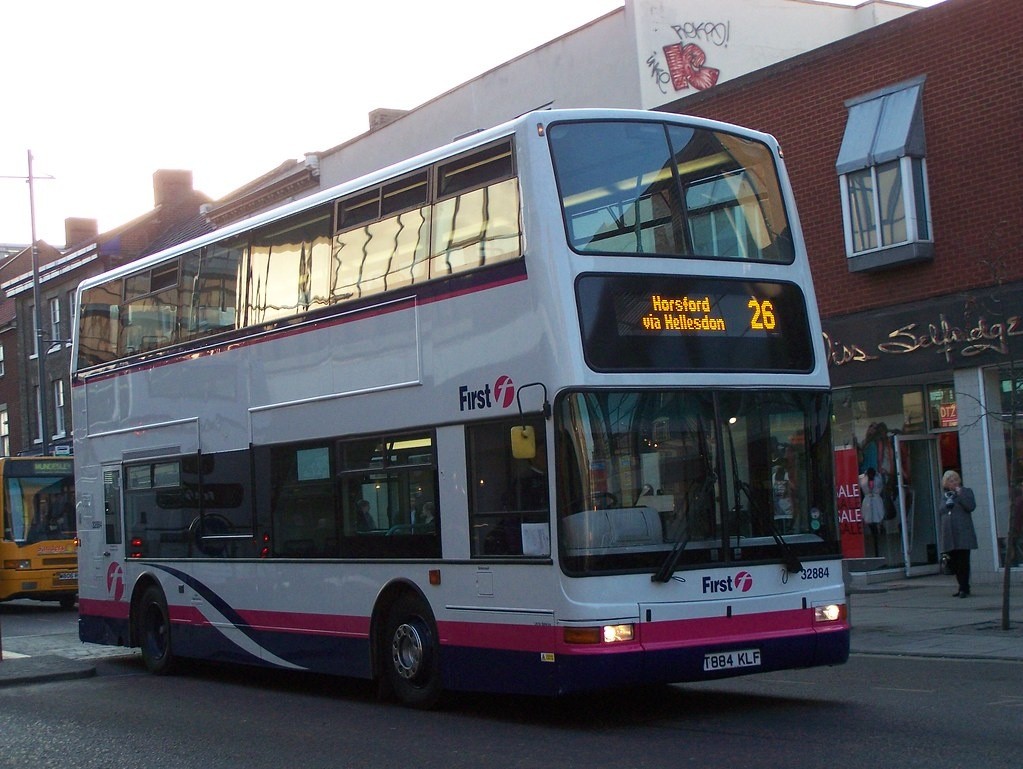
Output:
<box><xmin>940</xmin><ymin>552</ymin><xmax>955</xmax><ymax>575</ymax></box>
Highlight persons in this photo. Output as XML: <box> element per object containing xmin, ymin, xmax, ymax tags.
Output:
<box><xmin>859</xmin><ymin>468</ymin><xmax>885</xmax><ymax>558</ymax></box>
<box><xmin>502</xmin><ymin>440</ymin><xmax>550</xmax><ymax>524</ymax></box>
<box><xmin>939</xmin><ymin>470</ymin><xmax>979</xmax><ymax>598</ymax></box>
<box><xmin>410</xmin><ymin>492</ymin><xmax>435</xmax><ymax>533</ymax></box>
<box><xmin>772</xmin><ymin>458</ymin><xmax>797</xmax><ymax>519</ymax></box>
<box><xmin>358</xmin><ymin>500</ymin><xmax>376</xmax><ymax>529</ymax></box>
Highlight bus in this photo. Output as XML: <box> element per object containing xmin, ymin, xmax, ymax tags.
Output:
<box><xmin>0</xmin><ymin>456</ymin><xmax>76</xmax><ymax>611</ymax></box>
<box><xmin>69</xmin><ymin>108</ymin><xmax>857</xmax><ymax>710</ymax></box>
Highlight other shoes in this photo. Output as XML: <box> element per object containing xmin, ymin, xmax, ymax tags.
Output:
<box><xmin>952</xmin><ymin>591</ymin><xmax>969</xmax><ymax>598</ymax></box>
